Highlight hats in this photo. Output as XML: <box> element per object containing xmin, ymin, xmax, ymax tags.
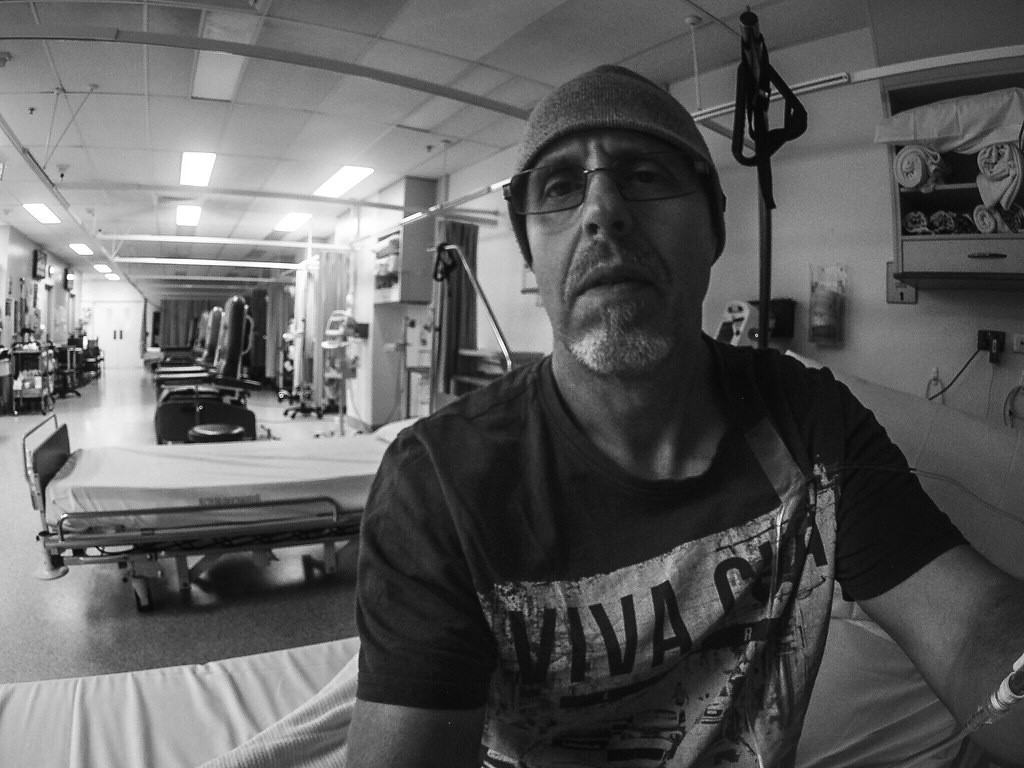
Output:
<box><xmin>505</xmin><ymin>66</ymin><xmax>726</xmax><ymax>277</ymax></box>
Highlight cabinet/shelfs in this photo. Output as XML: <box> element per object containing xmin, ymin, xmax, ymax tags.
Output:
<box><xmin>875</xmin><ymin>45</ymin><xmax>1023</xmax><ymax>281</ymax></box>
<box><xmin>374</xmin><ymin>219</ymin><xmax>434</xmax><ymax>305</ymax></box>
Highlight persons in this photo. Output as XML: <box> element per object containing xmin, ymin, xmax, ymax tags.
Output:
<box><xmin>345</xmin><ymin>65</ymin><xmax>1023</xmax><ymax>768</ymax></box>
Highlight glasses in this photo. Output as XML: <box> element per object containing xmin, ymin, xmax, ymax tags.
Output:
<box><xmin>504</xmin><ymin>149</ymin><xmax>713</xmax><ymax>217</ymax></box>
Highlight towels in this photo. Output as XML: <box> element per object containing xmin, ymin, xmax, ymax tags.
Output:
<box><xmin>904</xmin><ymin>208</ymin><xmax>929</xmax><ymax>233</ymax></box>
<box><xmin>892</xmin><ymin>143</ymin><xmax>954</xmax><ymax>192</ymax></box>
<box><xmin>928</xmin><ymin>208</ymin><xmax>972</xmax><ymax>233</ymax></box>
<box><xmin>971</xmin><ymin>202</ymin><xmax>1009</xmax><ymax>232</ymax></box>
<box><xmin>190</xmin><ymin>649</ymin><xmax>366</xmax><ymax>768</ymax></box>
<box><xmin>975</xmin><ymin>143</ymin><xmax>1024</xmax><ymax>212</ymax></box>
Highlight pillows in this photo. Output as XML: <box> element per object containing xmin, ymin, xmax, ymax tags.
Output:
<box><xmin>370</xmin><ymin>416</ymin><xmax>424</xmax><ymax>444</ymax></box>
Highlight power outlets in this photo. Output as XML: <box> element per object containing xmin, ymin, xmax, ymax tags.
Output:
<box><xmin>977</xmin><ymin>330</ymin><xmax>1005</xmax><ymax>352</ymax></box>
<box><xmin>1013</xmin><ymin>334</ymin><xmax>1024</xmax><ymax>353</ymax></box>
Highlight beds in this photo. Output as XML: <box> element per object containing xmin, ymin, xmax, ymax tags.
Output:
<box><xmin>1</xmin><ymin>633</ymin><xmax>361</xmax><ymax>766</ymax></box>
<box><xmin>46</xmin><ymin>418</ymin><xmax>433</xmax><ymax>609</ymax></box>
